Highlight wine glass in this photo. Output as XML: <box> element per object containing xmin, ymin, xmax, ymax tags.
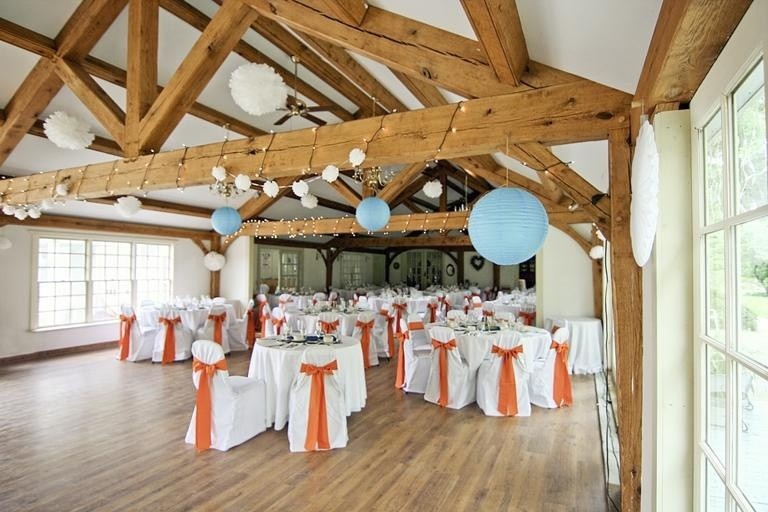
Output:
<box><xmin>291</xmin><ymin>319</ymin><xmax>342</xmax><ymax>344</ymax></box>
<box><xmin>294</xmin><ymin>298</ymin><xmax>353</xmax><ymax>308</ymax></box>
<box><xmin>439</xmin><ymin>312</ymin><xmax>524</xmax><ymax>333</ymax></box>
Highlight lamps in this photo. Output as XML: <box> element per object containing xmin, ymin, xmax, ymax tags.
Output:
<box><xmin>352</xmin><ymin>95</ymin><xmax>395</xmax><ymax>192</ymax></box>
<box><xmin>208</xmin><ymin>123</ymin><xmax>246</xmax><ymax>198</ymax></box>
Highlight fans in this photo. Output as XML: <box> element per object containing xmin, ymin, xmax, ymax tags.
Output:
<box><xmin>274</xmin><ymin>55</ymin><xmax>335</xmax><ymax>126</ymax></box>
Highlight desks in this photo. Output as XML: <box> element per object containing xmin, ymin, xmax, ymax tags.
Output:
<box><xmin>561</xmin><ymin>317</ymin><xmax>603</xmax><ymax>375</ymax></box>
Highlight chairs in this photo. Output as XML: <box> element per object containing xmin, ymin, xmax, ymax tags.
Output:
<box><xmin>288</xmin><ymin>344</ymin><xmax>350</xmax><ymax>452</ymax></box>
<box><xmin>184</xmin><ymin>340</ymin><xmax>266</xmax><ymax>452</ymax></box>
<box><xmin>476</xmin><ymin>331</ymin><xmax>531</xmax><ymax>418</ymax></box>
<box><xmin>424</xmin><ymin>326</ymin><xmax>476</xmax><ymax>410</ymax></box>
<box><xmin>528</xmin><ymin>327</ymin><xmax>573</xmax><ymax>409</ymax></box>
<box><xmin>395</xmin><ymin>319</ymin><xmax>432</xmax><ymax>394</ymax></box>
